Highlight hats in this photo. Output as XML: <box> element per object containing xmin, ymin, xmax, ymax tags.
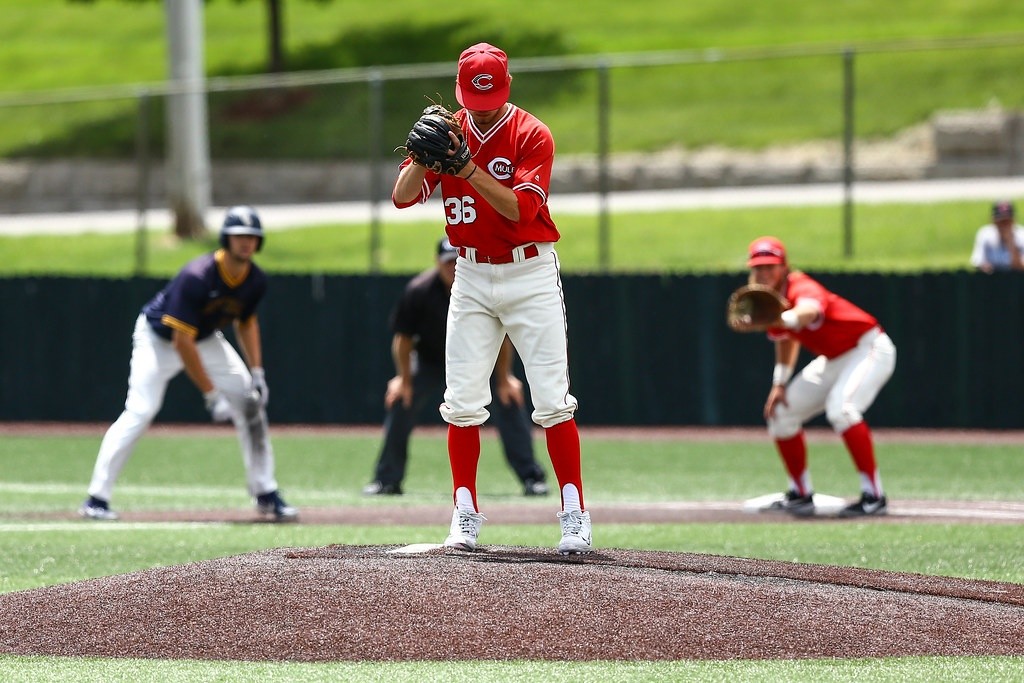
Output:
<box><xmin>748</xmin><ymin>236</ymin><xmax>786</xmax><ymax>267</ymax></box>
<box><xmin>992</xmin><ymin>201</ymin><xmax>1012</xmax><ymax>222</ymax></box>
<box><xmin>455</xmin><ymin>43</ymin><xmax>511</xmax><ymax>111</ymax></box>
<box><xmin>438</xmin><ymin>238</ymin><xmax>458</xmax><ymax>262</ymax></box>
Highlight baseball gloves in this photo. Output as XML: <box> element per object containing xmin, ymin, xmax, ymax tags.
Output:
<box><xmin>727</xmin><ymin>284</ymin><xmax>792</xmax><ymax>332</ymax></box>
<box><xmin>405</xmin><ymin>104</ymin><xmax>472</xmax><ymax>176</ymax></box>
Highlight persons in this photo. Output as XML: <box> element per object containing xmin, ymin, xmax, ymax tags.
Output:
<box><xmin>970</xmin><ymin>202</ymin><xmax>1024</xmax><ymax>274</ymax></box>
<box><xmin>391</xmin><ymin>41</ymin><xmax>592</xmax><ymax>553</ymax></box>
<box><xmin>82</xmin><ymin>206</ymin><xmax>297</xmax><ymax>519</ymax></box>
<box><xmin>748</xmin><ymin>235</ymin><xmax>898</xmax><ymax>519</ymax></box>
<box><xmin>359</xmin><ymin>238</ymin><xmax>551</xmax><ymax>496</ymax></box>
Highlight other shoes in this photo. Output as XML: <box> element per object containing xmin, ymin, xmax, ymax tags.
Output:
<box><xmin>524</xmin><ymin>482</ymin><xmax>548</xmax><ymax>495</ymax></box>
<box><xmin>363</xmin><ymin>482</ymin><xmax>402</xmax><ymax>495</ymax></box>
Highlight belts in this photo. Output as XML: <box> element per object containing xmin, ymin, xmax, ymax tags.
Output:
<box><xmin>459</xmin><ymin>244</ymin><xmax>538</xmax><ymax>264</ymax></box>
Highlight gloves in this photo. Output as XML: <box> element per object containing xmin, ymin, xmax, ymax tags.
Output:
<box><xmin>249</xmin><ymin>368</ymin><xmax>268</xmax><ymax>408</ymax></box>
<box><xmin>203</xmin><ymin>391</ymin><xmax>233</xmax><ymax>422</ymax></box>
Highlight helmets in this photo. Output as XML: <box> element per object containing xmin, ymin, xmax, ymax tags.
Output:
<box><xmin>219</xmin><ymin>206</ymin><xmax>264</xmax><ymax>252</ymax></box>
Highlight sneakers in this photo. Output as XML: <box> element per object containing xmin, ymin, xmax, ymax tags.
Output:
<box><xmin>444</xmin><ymin>507</ymin><xmax>488</xmax><ymax>551</ymax></box>
<box><xmin>77</xmin><ymin>495</ymin><xmax>119</xmax><ymax>520</ymax></box>
<box><xmin>839</xmin><ymin>491</ymin><xmax>887</xmax><ymax>518</ymax></box>
<box><xmin>556</xmin><ymin>509</ymin><xmax>592</xmax><ymax>555</ymax></box>
<box><xmin>759</xmin><ymin>489</ymin><xmax>815</xmax><ymax>517</ymax></box>
<box><xmin>256</xmin><ymin>489</ymin><xmax>298</xmax><ymax>519</ymax></box>
